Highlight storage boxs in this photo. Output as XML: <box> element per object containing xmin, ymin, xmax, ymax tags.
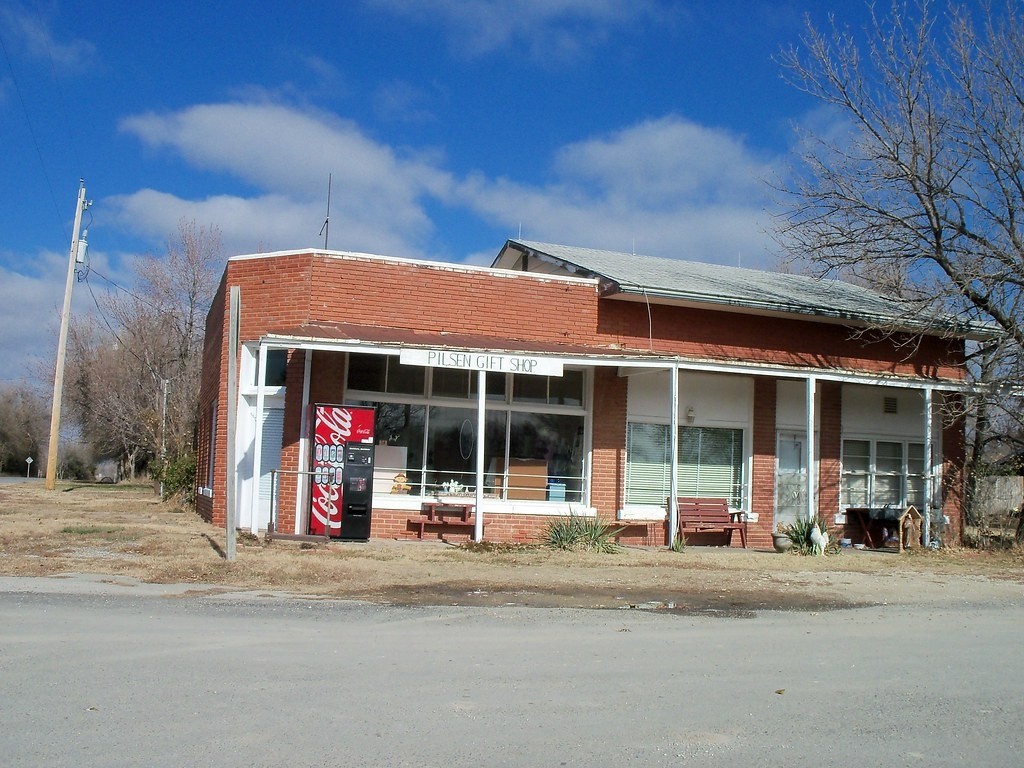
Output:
<box><xmin>547</xmin><ymin>483</ymin><xmax>566</xmax><ymax>502</ymax></box>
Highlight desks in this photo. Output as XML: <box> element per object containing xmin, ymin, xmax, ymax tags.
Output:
<box><xmin>846</xmin><ymin>508</ymin><xmax>907</xmax><ymax>548</ymax></box>
<box><xmin>612</xmin><ymin>520</ymin><xmax>658</xmax><ymax>548</ymax></box>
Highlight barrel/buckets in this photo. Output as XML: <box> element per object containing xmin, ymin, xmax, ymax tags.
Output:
<box><xmin>840</xmin><ymin>538</ymin><xmax>852</xmax><ymax>548</ymax></box>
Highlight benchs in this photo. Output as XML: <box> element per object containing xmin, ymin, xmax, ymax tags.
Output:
<box><xmin>667</xmin><ymin>497</ymin><xmax>748</xmax><ymax>549</ymax></box>
<box><xmin>410</xmin><ymin>503</ymin><xmax>492</xmax><ymax>539</ymax></box>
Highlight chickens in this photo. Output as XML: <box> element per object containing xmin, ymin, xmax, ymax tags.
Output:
<box><xmin>810</xmin><ymin>521</ymin><xmax>829</xmax><ymax>556</ymax></box>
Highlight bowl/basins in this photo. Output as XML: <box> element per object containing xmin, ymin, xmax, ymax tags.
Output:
<box><xmin>853</xmin><ymin>543</ymin><xmax>865</xmax><ymax>550</ymax></box>
